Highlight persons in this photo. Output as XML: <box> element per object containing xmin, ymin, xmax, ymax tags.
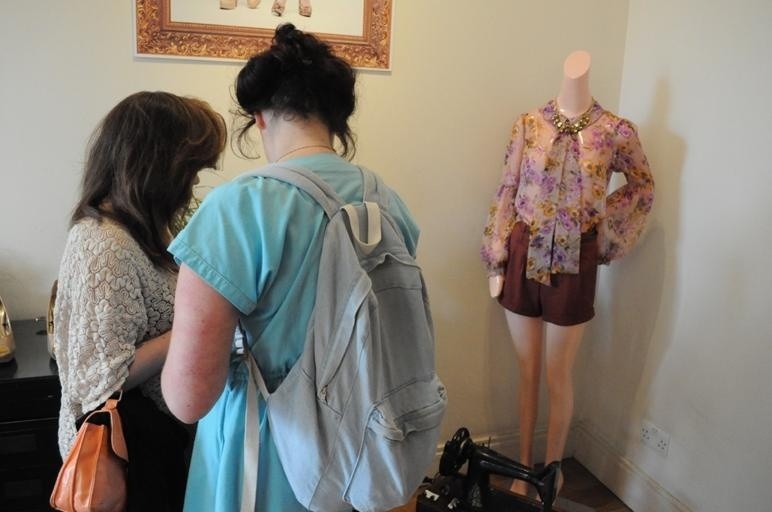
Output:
<box><xmin>50</xmin><ymin>91</ymin><xmax>228</xmax><ymax>512</ymax></box>
<box><xmin>480</xmin><ymin>45</ymin><xmax>658</xmax><ymax>506</ymax></box>
<box><xmin>158</xmin><ymin>18</ymin><xmax>446</xmax><ymax>512</ymax></box>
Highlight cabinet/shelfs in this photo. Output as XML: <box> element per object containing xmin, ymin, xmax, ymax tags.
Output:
<box><xmin>1</xmin><ymin>385</ymin><xmax>68</xmax><ymax>511</ymax></box>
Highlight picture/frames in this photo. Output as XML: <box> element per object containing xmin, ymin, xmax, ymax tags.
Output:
<box><xmin>133</xmin><ymin>0</ymin><xmax>394</xmax><ymax>73</ymax></box>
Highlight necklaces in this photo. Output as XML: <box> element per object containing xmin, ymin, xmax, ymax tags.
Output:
<box><xmin>266</xmin><ymin>143</ymin><xmax>337</xmax><ymax>164</ymax></box>
<box><xmin>551</xmin><ymin>99</ymin><xmax>595</xmax><ymax>136</ymax></box>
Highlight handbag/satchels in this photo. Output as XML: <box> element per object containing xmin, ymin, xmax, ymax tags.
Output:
<box><xmin>49</xmin><ymin>398</ymin><xmax>131</xmax><ymax>510</ymax></box>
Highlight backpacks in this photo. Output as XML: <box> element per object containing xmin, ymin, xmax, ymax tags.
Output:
<box><xmin>221</xmin><ymin>160</ymin><xmax>447</xmax><ymax>511</ymax></box>
